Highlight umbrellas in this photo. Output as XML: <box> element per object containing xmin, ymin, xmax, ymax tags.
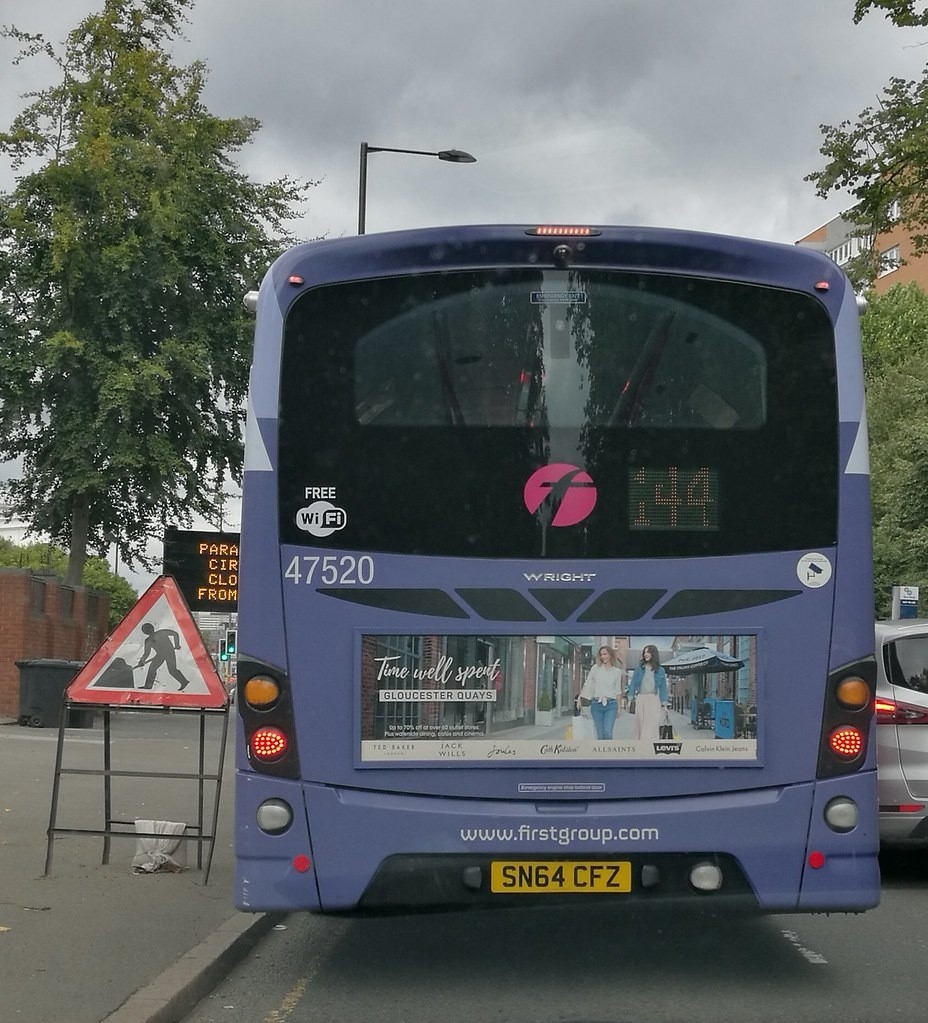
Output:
<box><xmin>659</xmin><ymin>646</ymin><xmax>745</xmax><ymax>702</ymax></box>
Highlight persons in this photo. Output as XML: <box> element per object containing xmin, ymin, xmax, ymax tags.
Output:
<box><xmin>625</xmin><ymin>645</ymin><xmax>670</xmax><ymax>741</ymax></box>
<box><xmin>575</xmin><ymin>647</ymin><xmax>625</xmax><ymax>741</ymax></box>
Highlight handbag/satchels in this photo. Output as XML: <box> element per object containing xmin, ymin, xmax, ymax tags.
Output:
<box><xmin>629</xmin><ymin>699</ymin><xmax>635</xmax><ymax>714</ymax></box>
<box><xmin>659</xmin><ymin>715</ymin><xmax>673</xmax><ymax>739</ymax></box>
<box><xmin>571</xmin><ymin>708</ymin><xmax>595</xmax><ymax>742</ymax></box>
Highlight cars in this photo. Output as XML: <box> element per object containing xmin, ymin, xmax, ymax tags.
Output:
<box><xmin>873</xmin><ymin>619</ymin><xmax>928</xmax><ymax>861</ymax></box>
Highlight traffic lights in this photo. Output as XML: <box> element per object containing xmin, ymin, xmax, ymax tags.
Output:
<box><xmin>219</xmin><ymin>638</ymin><xmax>228</xmax><ymax>662</ymax></box>
<box><xmin>226</xmin><ymin>630</ymin><xmax>236</xmax><ymax>655</ymax></box>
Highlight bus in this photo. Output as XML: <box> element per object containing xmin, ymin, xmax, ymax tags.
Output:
<box><xmin>237</xmin><ymin>222</ymin><xmax>881</xmax><ymax>916</ymax></box>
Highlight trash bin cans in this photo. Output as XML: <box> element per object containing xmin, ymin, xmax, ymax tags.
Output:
<box><xmin>14</xmin><ymin>658</ymin><xmax>70</xmax><ymax>729</ymax></box>
<box><xmin>68</xmin><ymin>661</ymin><xmax>93</xmax><ymax>729</ymax></box>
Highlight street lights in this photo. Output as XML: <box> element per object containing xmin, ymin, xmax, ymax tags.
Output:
<box><xmin>359</xmin><ymin>142</ymin><xmax>476</xmax><ymax>235</ymax></box>
